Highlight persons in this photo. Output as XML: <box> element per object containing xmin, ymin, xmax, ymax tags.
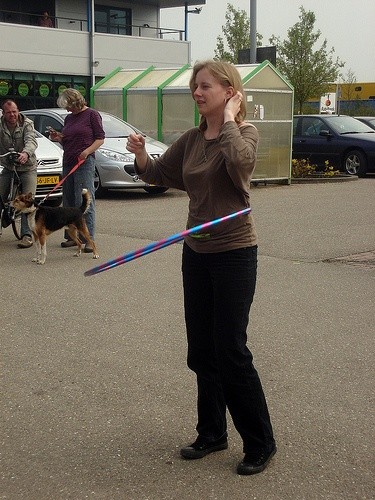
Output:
<box><xmin>43</xmin><ymin>89</ymin><xmax>105</xmax><ymax>252</ymax></box>
<box><xmin>126</xmin><ymin>60</ymin><xmax>275</xmax><ymax>477</ymax></box>
<box><xmin>0</xmin><ymin>99</ymin><xmax>39</xmax><ymax>248</ymax></box>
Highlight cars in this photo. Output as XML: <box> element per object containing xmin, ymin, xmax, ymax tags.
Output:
<box><xmin>18</xmin><ymin>109</ymin><xmax>171</xmax><ymax>198</ymax></box>
<box><xmin>0</xmin><ymin>108</ymin><xmax>65</xmax><ymax>208</ymax></box>
<box><xmin>351</xmin><ymin>116</ymin><xmax>375</xmax><ymax>130</ymax></box>
<box><xmin>292</xmin><ymin>114</ymin><xmax>375</xmax><ymax>177</ymax></box>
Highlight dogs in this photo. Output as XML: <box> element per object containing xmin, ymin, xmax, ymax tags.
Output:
<box><xmin>8</xmin><ymin>188</ymin><xmax>100</xmax><ymax>265</ymax></box>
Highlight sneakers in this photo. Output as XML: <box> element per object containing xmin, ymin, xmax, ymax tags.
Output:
<box><xmin>237</xmin><ymin>444</ymin><xmax>277</xmax><ymax>475</ymax></box>
<box><xmin>61</xmin><ymin>239</ymin><xmax>77</xmax><ymax>247</ymax></box>
<box><xmin>180</xmin><ymin>435</ymin><xmax>228</xmax><ymax>459</ymax></box>
<box><xmin>18</xmin><ymin>236</ymin><xmax>33</xmax><ymax>248</ymax></box>
<box><xmin>84</xmin><ymin>242</ymin><xmax>93</xmax><ymax>252</ymax></box>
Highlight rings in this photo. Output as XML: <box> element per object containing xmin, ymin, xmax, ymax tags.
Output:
<box><xmin>21</xmin><ymin>161</ymin><xmax>24</xmax><ymax>163</ymax></box>
<box><xmin>127</xmin><ymin>141</ymin><xmax>131</xmax><ymax>146</ymax></box>
